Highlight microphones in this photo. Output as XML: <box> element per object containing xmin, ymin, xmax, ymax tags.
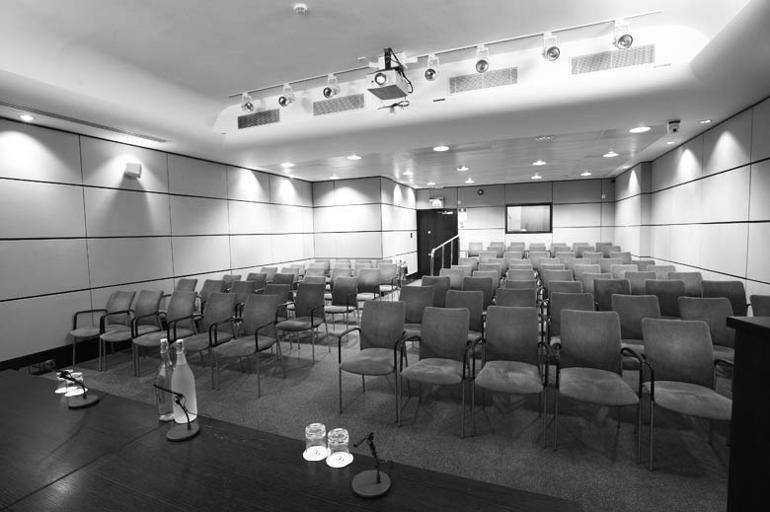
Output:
<box><xmin>31</xmin><ymin>364</ymin><xmax>99</xmax><ymax>410</ymax></box>
<box><xmin>351</xmin><ymin>431</ymin><xmax>391</xmax><ymax>498</ymax></box>
<box><xmin>151</xmin><ymin>382</ymin><xmax>201</xmax><ymax>442</ymax></box>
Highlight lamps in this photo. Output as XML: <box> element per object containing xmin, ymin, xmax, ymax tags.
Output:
<box><xmin>322</xmin><ymin>76</ymin><xmax>341</xmax><ymax>97</ymax></box>
<box><xmin>423</xmin><ymin>56</ymin><xmax>439</xmax><ymax>82</ymax></box>
<box><xmin>473</xmin><ymin>47</ymin><xmax>490</xmax><ymax>73</ymax></box>
<box><xmin>610</xmin><ymin>19</ymin><xmax>637</xmax><ymax>50</ymax></box>
<box><xmin>239</xmin><ymin>94</ymin><xmax>256</xmax><ymax>114</ymax></box>
<box><xmin>278</xmin><ymin>85</ymin><xmax>298</xmax><ymax>105</ymax></box>
<box><xmin>540</xmin><ymin>35</ymin><xmax>562</xmax><ymax>61</ymax></box>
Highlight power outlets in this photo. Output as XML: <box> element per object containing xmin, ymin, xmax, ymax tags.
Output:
<box><xmin>20</xmin><ymin>360</ymin><xmax>52</xmax><ymax>375</ymax></box>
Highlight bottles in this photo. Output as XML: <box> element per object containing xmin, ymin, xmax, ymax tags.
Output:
<box><xmin>171</xmin><ymin>339</ymin><xmax>198</xmax><ymax>424</ymax></box>
<box><xmin>154</xmin><ymin>338</ymin><xmax>174</xmax><ymax>423</ymax></box>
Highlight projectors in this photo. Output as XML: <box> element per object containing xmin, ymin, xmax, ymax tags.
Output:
<box><xmin>365</xmin><ymin>68</ymin><xmax>409</xmax><ymax>101</ymax></box>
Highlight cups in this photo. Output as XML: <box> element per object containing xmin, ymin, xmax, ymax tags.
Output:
<box><xmin>57</xmin><ymin>370</ymin><xmax>73</xmax><ymax>394</ymax></box>
<box><xmin>329</xmin><ymin>428</ymin><xmax>349</xmax><ymax>467</ymax></box>
<box><xmin>66</xmin><ymin>372</ymin><xmax>83</xmax><ymax>397</ymax></box>
<box><xmin>305</xmin><ymin>423</ymin><xmax>328</xmax><ymax>462</ymax></box>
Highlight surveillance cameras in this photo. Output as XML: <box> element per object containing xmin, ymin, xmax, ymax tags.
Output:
<box><xmin>477</xmin><ymin>189</ymin><xmax>484</xmax><ymax>195</ymax></box>
<box><xmin>669</xmin><ymin>125</ymin><xmax>680</xmax><ymax>137</ymax></box>
